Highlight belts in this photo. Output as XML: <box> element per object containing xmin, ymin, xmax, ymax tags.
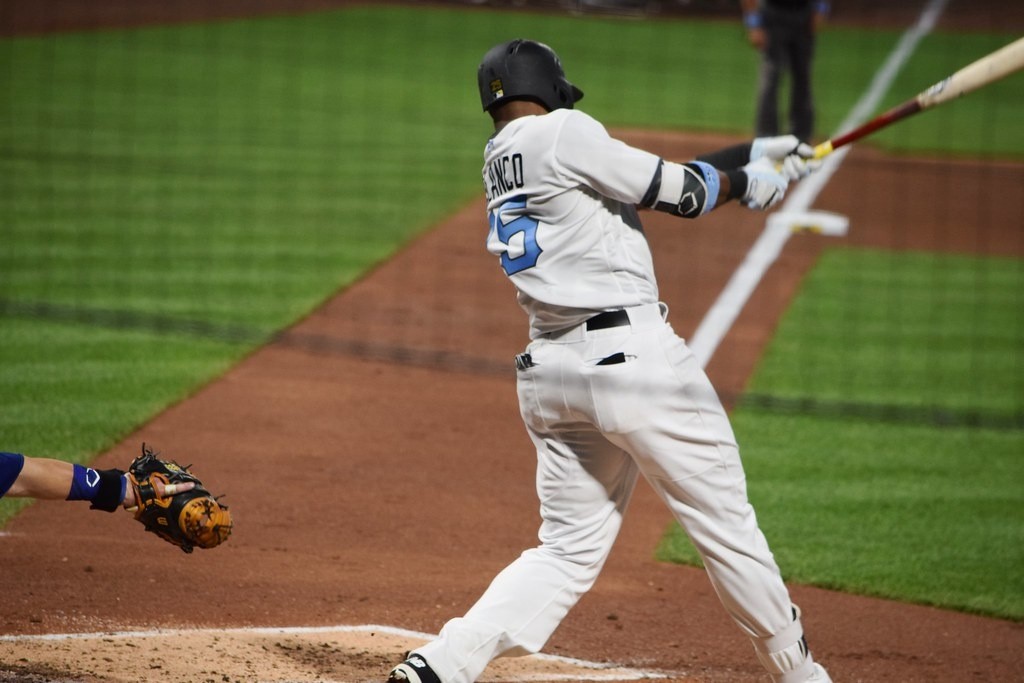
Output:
<box><xmin>542</xmin><ymin>310</ymin><xmax>630</xmax><ymax>333</ymax></box>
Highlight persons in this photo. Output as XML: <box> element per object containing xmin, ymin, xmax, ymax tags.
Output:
<box><xmin>390</xmin><ymin>41</ymin><xmax>832</xmax><ymax>683</ymax></box>
<box><xmin>0</xmin><ymin>451</ymin><xmax>234</xmax><ymax>555</ymax></box>
<box><xmin>742</xmin><ymin>0</ymin><xmax>831</xmax><ymax>143</ymax></box>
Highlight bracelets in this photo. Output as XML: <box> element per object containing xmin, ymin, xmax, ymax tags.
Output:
<box><xmin>746</xmin><ymin>15</ymin><xmax>762</xmax><ymax>27</ymax></box>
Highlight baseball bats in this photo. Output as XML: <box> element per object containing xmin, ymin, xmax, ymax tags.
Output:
<box><xmin>775</xmin><ymin>35</ymin><xmax>1024</xmax><ymax>176</ymax></box>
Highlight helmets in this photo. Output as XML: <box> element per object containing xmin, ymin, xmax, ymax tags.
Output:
<box><xmin>477</xmin><ymin>39</ymin><xmax>586</xmax><ymax>111</ymax></box>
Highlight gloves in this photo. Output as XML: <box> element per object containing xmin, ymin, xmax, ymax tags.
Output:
<box><xmin>748</xmin><ymin>134</ymin><xmax>822</xmax><ymax>184</ymax></box>
<box><xmin>736</xmin><ymin>156</ymin><xmax>790</xmax><ymax>213</ymax></box>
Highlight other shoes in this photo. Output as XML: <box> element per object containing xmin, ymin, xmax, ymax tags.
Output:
<box><xmin>387</xmin><ymin>654</ymin><xmax>442</xmax><ymax>683</ymax></box>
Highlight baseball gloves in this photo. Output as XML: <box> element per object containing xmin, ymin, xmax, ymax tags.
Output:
<box><xmin>128</xmin><ymin>452</ymin><xmax>234</xmax><ymax>554</ymax></box>
